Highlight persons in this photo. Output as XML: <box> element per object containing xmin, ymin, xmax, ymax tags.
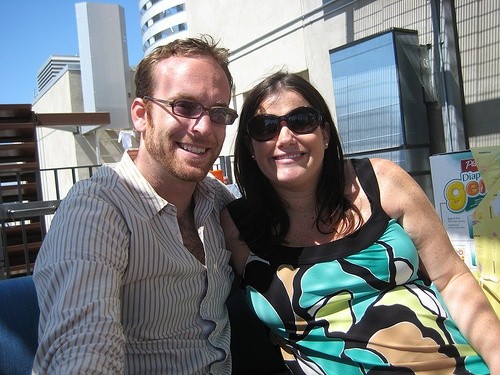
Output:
<box><xmin>32</xmin><ymin>33</ymin><xmax>241</xmax><ymax>375</ymax></box>
<box><xmin>221</xmin><ymin>70</ymin><xmax>500</xmax><ymax>375</ymax></box>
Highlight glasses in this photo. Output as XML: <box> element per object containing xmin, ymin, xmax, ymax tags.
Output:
<box><xmin>141</xmin><ymin>95</ymin><xmax>239</xmax><ymax>125</ymax></box>
<box><xmin>245</xmin><ymin>107</ymin><xmax>325</xmax><ymax>142</ymax></box>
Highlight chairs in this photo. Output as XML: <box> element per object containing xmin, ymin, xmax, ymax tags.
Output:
<box><xmin>1</xmin><ymin>272</ymin><xmax>289</xmax><ymax>374</ymax></box>
